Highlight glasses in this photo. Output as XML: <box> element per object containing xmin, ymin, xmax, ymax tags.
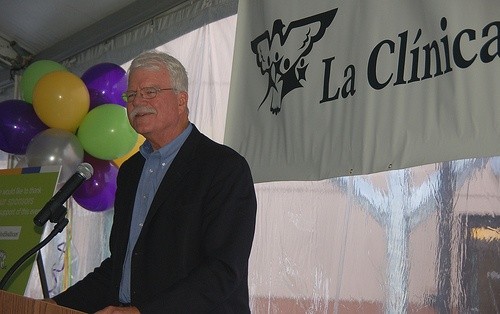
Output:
<box><xmin>120</xmin><ymin>87</ymin><xmax>178</xmax><ymax>102</ymax></box>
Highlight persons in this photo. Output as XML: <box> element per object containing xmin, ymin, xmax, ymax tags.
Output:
<box><xmin>37</xmin><ymin>50</ymin><xmax>257</xmax><ymax>314</ymax></box>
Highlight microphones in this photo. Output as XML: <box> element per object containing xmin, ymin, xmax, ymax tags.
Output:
<box><xmin>34</xmin><ymin>163</ymin><xmax>94</xmax><ymax>227</ymax></box>
